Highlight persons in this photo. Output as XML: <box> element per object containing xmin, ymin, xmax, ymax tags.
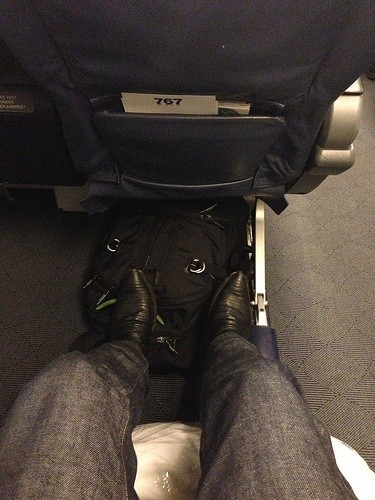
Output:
<box><xmin>0</xmin><ymin>268</ymin><xmax>359</xmax><ymax>500</ymax></box>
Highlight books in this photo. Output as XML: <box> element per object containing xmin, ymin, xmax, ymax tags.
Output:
<box><xmin>216</xmin><ymin>99</ymin><xmax>250</xmax><ymax>115</ymax></box>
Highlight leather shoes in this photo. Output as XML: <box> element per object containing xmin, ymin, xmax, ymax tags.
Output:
<box><xmin>110</xmin><ymin>269</ymin><xmax>157</xmax><ymax>367</ymax></box>
<box><xmin>207</xmin><ymin>271</ymin><xmax>252</xmax><ymax>342</ymax></box>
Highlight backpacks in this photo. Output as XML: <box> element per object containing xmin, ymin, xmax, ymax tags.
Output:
<box><xmin>84</xmin><ymin>196</ymin><xmax>252</xmax><ymax>355</ymax></box>
